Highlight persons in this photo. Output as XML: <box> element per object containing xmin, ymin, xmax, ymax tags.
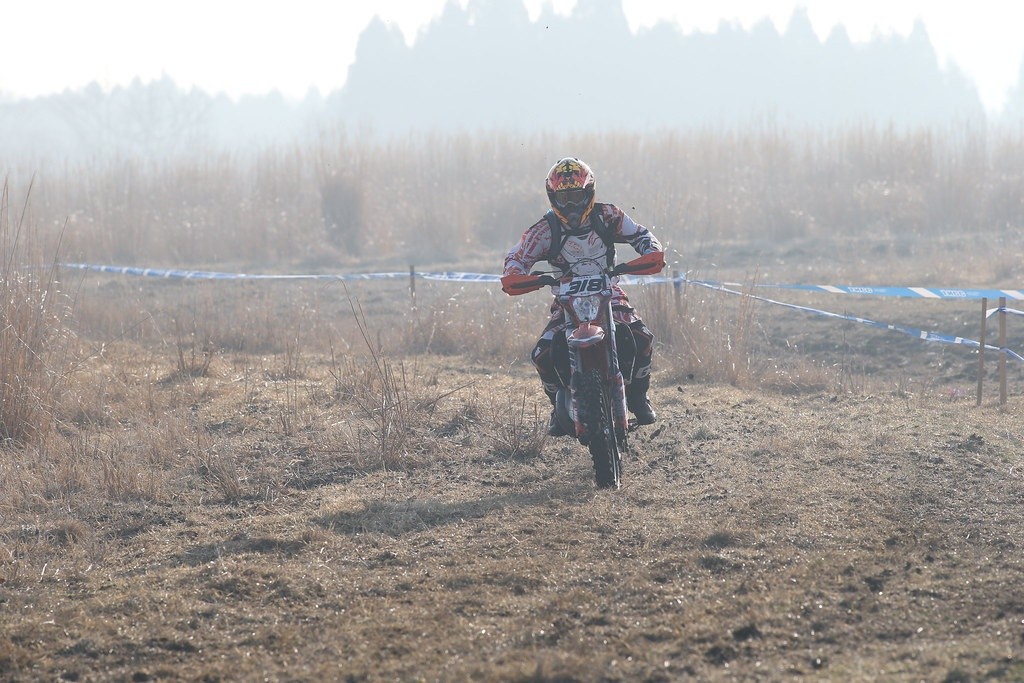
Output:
<box><xmin>502</xmin><ymin>158</ymin><xmax>665</xmax><ymax>437</ymax></box>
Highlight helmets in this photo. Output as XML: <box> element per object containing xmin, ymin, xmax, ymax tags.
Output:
<box><xmin>545</xmin><ymin>157</ymin><xmax>595</xmax><ymax>228</ymax></box>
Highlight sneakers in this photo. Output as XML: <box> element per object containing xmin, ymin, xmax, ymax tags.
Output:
<box><xmin>628</xmin><ymin>398</ymin><xmax>656</xmax><ymax>424</ymax></box>
<box><xmin>548</xmin><ymin>416</ymin><xmax>566</xmax><ymax>436</ymax></box>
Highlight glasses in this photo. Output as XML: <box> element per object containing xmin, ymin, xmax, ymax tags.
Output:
<box><xmin>549</xmin><ymin>190</ymin><xmax>587</xmax><ymax>208</ymax></box>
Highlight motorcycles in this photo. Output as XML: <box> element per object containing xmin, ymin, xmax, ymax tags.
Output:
<box><xmin>500</xmin><ymin>252</ymin><xmax>667</xmax><ymax>490</ymax></box>
